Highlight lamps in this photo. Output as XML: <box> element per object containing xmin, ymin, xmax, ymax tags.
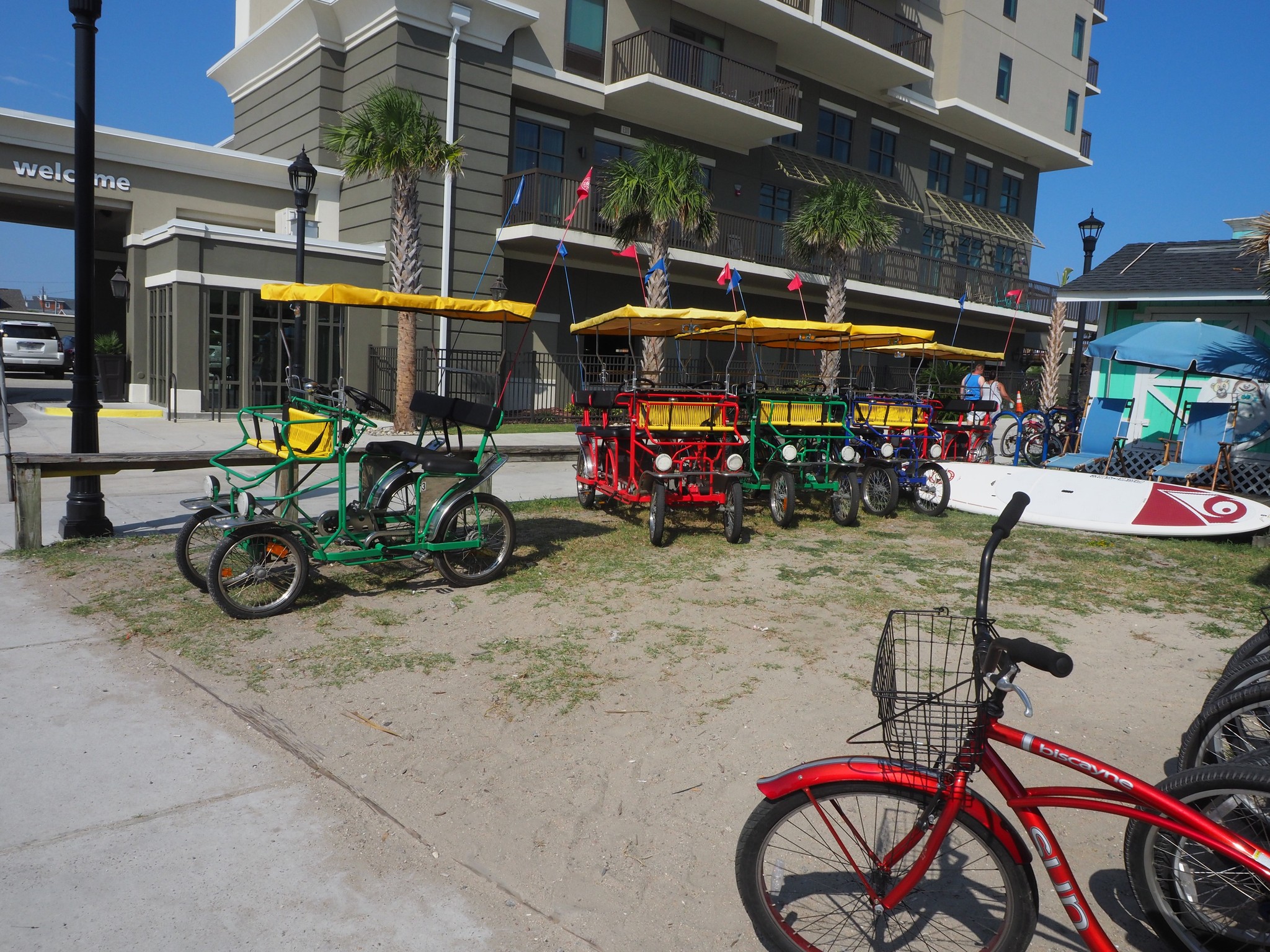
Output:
<box><xmin>733</xmin><ymin>183</ymin><xmax>742</xmax><ymax>196</ymax></box>
<box><xmin>988</xmin><ymin>250</ymin><xmax>996</xmax><ymax>259</ymax></box>
<box><xmin>905</xmin><ymin>228</ymin><xmax>911</xmax><ymax>234</ymax></box>
<box><xmin>489</xmin><ymin>274</ymin><xmax>509</xmax><ymax>301</ymax></box>
<box><xmin>578</xmin><ymin>145</ymin><xmax>587</xmax><ymax>159</ymax></box>
<box><xmin>1018</xmin><ymin>257</ymin><xmax>1026</xmax><ymax>269</ymax></box>
<box><xmin>109</xmin><ymin>265</ymin><xmax>131</xmax><ymax>302</ymax></box>
<box><xmin>951</xmin><ymin>240</ymin><xmax>957</xmax><ymax>248</ymax></box>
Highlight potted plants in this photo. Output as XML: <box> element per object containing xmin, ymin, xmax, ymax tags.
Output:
<box><xmin>95</xmin><ymin>328</ymin><xmax>126</xmax><ymax>402</ymax></box>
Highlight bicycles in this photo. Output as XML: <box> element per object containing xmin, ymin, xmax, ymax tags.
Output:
<box><xmin>729</xmin><ymin>489</ymin><xmax>1270</xmax><ymax>952</ymax></box>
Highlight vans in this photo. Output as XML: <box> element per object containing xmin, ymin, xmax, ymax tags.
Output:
<box><xmin>0</xmin><ymin>319</ymin><xmax>66</xmax><ymax>380</ymax></box>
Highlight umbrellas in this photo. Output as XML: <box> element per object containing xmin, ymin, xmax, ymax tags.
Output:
<box><xmin>1083</xmin><ymin>319</ymin><xmax>1270</xmax><ymax>439</ymax></box>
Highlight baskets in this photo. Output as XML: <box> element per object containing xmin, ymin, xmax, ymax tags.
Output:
<box><xmin>870</xmin><ymin>606</ymin><xmax>996</xmax><ymax>774</ymax></box>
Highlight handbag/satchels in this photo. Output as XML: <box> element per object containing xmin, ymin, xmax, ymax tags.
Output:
<box><xmin>1000</xmin><ymin>403</ymin><xmax>1003</xmax><ymax>410</ymax></box>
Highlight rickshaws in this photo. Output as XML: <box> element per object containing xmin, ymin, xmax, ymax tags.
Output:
<box><xmin>568</xmin><ymin>304</ymin><xmax>1001</xmax><ymax>546</ymax></box>
<box><xmin>172</xmin><ymin>283</ymin><xmax>537</xmax><ymax>621</ymax></box>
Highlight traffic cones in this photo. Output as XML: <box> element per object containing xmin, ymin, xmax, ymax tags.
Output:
<box><xmin>1015</xmin><ymin>390</ymin><xmax>1024</xmax><ymax>416</ymax></box>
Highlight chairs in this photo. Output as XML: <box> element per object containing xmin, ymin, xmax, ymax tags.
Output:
<box><xmin>1145</xmin><ymin>399</ymin><xmax>1240</xmax><ymax>494</ymax></box>
<box><xmin>965</xmin><ymin>280</ymin><xmax>1031</xmax><ymax>311</ymax></box>
<box><xmin>726</xmin><ymin>234</ymin><xmax>757</xmax><ymax>264</ymax></box>
<box><xmin>1040</xmin><ymin>394</ymin><xmax>1137</xmax><ymax>478</ymax></box>
<box><xmin>710</xmin><ymin>79</ymin><xmax>738</xmax><ymax>100</ymax></box>
<box><xmin>748</xmin><ymin>90</ymin><xmax>775</xmax><ymax>113</ymax></box>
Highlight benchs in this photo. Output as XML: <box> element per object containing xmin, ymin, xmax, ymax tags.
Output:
<box><xmin>364</xmin><ymin>389</ymin><xmax>505</xmax><ymax>476</ymax></box>
<box><xmin>589</xmin><ymin>390</ymin><xmax>705</xmax><ymax>438</ymax></box>
<box><xmin>246</xmin><ymin>405</ymin><xmax>338</xmax><ymax>462</ymax></box>
<box><xmin>928</xmin><ymin>398</ymin><xmax>975</xmax><ymax>432</ymax></box>
<box><xmin>728</xmin><ymin>392</ymin><xmax>929</xmax><ymax>429</ymax></box>
<box><xmin>571</xmin><ymin>389</ymin><xmax>644</xmax><ymax>435</ymax></box>
<box><xmin>938</xmin><ymin>399</ymin><xmax>999</xmax><ymax>434</ymax></box>
<box><xmin>636</xmin><ymin>398</ymin><xmax>735</xmax><ymax>431</ymax></box>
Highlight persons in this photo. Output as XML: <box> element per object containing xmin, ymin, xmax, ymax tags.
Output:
<box><xmin>961</xmin><ymin>364</ymin><xmax>985</xmax><ymax>426</ymax></box>
<box><xmin>980</xmin><ymin>371</ymin><xmax>1015</xmax><ymax>438</ymax></box>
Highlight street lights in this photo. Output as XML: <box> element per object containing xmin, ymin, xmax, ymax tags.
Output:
<box><xmin>1063</xmin><ymin>208</ymin><xmax>1106</xmax><ymax>452</ymax></box>
<box><xmin>285</xmin><ymin>144</ymin><xmax>318</xmax><ymax>408</ymax></box>
